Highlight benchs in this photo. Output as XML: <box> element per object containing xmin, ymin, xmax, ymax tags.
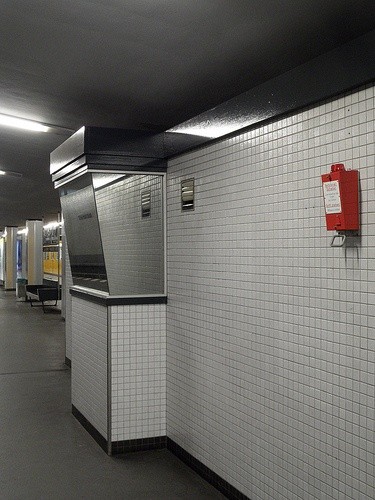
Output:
<box><xmin>25</xmin><ymin>279</ymin><xmax>62</xmax><ymax>313</ymax></box>
